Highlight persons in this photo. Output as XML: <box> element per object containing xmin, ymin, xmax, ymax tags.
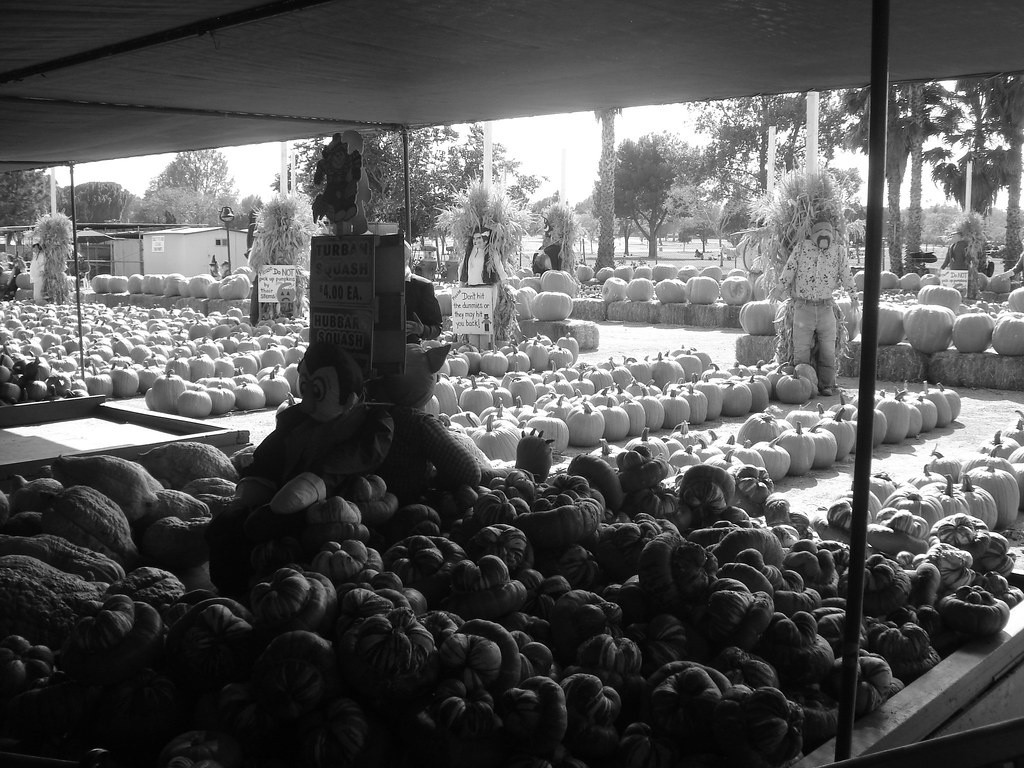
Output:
<box><xmin>0</xmin><ymin>254</ymin><xmax>28</xmax><ymax>301</ymax></box>
<box><xmin>403</xmin><ymin>240</ymin><xmax>442</xmax><ymax>343</ymax></box>
<box><xmin>78</xmin><ymin>257</ymin><xmax>89</xmax><ymax>284</ymax></box>
<box><xmin>30</xmin><ymin>243</ymin><xmax>45</xmax><ymax>301</ymax></box>
<box><xmin>995</xmin><ymin>237</ymin><xmax>1024</xmax><ymax>286</ymax></box>
<box><xmin>736</xmin><ymin>221</ymin><xmax>764</xmax><ymax>283</ymax></box>
<box><xmin>532</xmin><ymin>225</ymin><xmax>562</xmax><ymax>277</ymax></box>
<box><xmin>209</xmin><ymin>255</ymin><xmax>232</xmax><ymax>280</ymax></box>
<box><xmin>767</xmin><ymin>216</ymin><xmax>860</xmax><ymax>395</ymax></box>
<box><xmin>459</xmin><ymin>228</ymin><xmax>499</xmax><ymax>287</ymax></box>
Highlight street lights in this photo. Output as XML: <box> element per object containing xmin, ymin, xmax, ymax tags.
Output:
<box><xmin>218</xmin><ymin>207</ymin><xmax>234</xmax><ymax>276</ymax></box>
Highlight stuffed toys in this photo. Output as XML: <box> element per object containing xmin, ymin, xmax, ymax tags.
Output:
<box><xmin>379</xmin><ymin>343</ymin><xmax>481</xmax><ymax>506</ymax></box>
<box><xmin>235</xmin><ymin>342</ymin><xmax>394</xmax><ymax>533</ymax></box>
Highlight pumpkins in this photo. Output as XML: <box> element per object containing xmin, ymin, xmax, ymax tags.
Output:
<box><xmin>0</xmin><ymin>265</ymin><xmax>1024</xmax><ymax>768</ymax></box>
